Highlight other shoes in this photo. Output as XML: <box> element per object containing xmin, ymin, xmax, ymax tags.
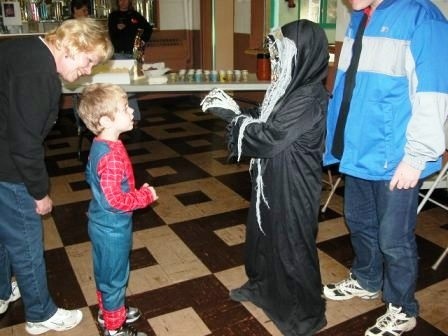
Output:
<box><xmin>230</xmin><ymin>285</ymin><xmax>253</xmax><ymax>302</ymax></box>
<box><xmin>102</xmin><ymin>325</ymin><xmax>148</xmax><ymax>336</ymax></box>
<box><xmin>97</xmin><ymin>307</ymin><xmax>141</xmax><ymax>324</ymax></box>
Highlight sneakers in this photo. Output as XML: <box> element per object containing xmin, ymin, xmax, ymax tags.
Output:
<box><xmin>365</xmin><ymin>303</ymin><xmax>417</xmax><ymax>336</ymax></box>
<box><xmin>25</xmin><ymin>309</ymin><xmax>82</xmax><ymax>333</ymax></box>
<box><xmin>0</xmin><ymin>280</ymin><xmax>21</xmax><ymax>315</ymax></box>
<box><xmin>323</xmin><ymin>273</ymin><xmax>381</xmax><ymax>300</ymax></box>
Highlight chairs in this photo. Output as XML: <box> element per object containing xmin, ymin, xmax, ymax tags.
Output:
<box><xmin>317</xmin><ymin>119</ymin><xmax>448</xmax><ymax>270</ymax></box>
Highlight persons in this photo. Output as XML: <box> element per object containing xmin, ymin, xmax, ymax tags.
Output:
<box><xmin>200</xmin><ymin>19</ymin><xmax>331</xmax><ymax>336</ymax></box>
<box><xmin>78</xmin><ymin>83</ymin><xmax>162</xmax><ymax>336</ymax></box>
<box><xmin>0</xmin><ymin>17</ymin><xmax>115</xmax><ymax>335</ymax></box>
<box><xmin>108</xmin><ymin>0</ymin><xmax>153</xmax><ymax>59</ymax></box>
<box><xmin>67</xmin><ymin>0</ymin><xmax>90</xmax><ymax>19</ymax></box>
<box><xmin>322</xmin><ymin>0</ymin><xmax>448</xmax><ymax>336</ymax></box>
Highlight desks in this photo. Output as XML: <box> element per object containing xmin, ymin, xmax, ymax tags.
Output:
<box><xmin>61</xmin><ymin>72</ymin><xmax>270</xmax><ymax>160</ymax></box>
